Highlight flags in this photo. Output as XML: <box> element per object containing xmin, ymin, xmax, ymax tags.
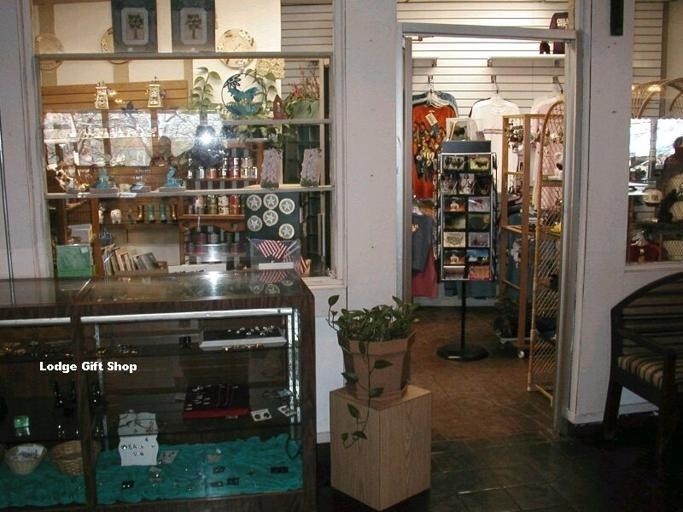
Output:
<box><xmin>250</xmin><ymin>268</ymin><xmax>295</xmax><ymax>283</ymax></box>
<box><xmin>249</xmin><ymin>238</ymin><xmax>300</xmax><ymax>262</ymax></box>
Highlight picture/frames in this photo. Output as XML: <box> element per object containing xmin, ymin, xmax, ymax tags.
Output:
<box><xmin>110</xmin><ymin>0</ymin><xmax>215</xmax><ymax>54</ymax></box>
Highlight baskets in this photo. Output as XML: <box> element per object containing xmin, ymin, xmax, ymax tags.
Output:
<box><xmin>3</xmin><ymin>443</ymin><xmax>48</xmax><ymax>475</ymax></box>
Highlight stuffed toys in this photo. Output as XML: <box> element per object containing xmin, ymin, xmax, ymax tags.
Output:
<box><xmin>510</xmin><ymin>239</ymin><xmax>522</xmax><ymax>266</ymax></box>
<box><xmin>545</xmin><ymin>151</ymin><xmax>563</xmax><ymax>182</ymax></box>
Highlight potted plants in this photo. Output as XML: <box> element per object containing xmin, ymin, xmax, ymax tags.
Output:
<box><xmin>324</xmin><ymin>293</ymin><xmax>420</xmax><ymax>448</ymax></box>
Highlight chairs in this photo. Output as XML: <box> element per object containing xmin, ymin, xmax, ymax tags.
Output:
<box><xmin>599</xmin><ymin>271</ymin><xmax>683</xmax><ymax>480</ymax></box>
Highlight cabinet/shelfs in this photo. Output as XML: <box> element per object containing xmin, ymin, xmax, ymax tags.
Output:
<box><xmin>432</xmin><ymin>114</ymin><xmax>565</xmax><ymax>362</ymax></box>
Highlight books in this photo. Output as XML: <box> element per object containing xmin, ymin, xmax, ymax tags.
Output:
<box><xmin>106</xmin><ymin>247</ymin><xmax>169</xmax><ymax>273</ymax></box>
<box><xmin>113</xmin><ymin>274</ymin><xmax>169</xmax><ymax>283</ymax></box>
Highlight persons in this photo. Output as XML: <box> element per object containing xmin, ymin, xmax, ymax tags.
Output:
<box><xmin>443</xmin><ymin>154</ymin><xmax>491</xmax><ymax>281</ymax></box>
<box><xmin>662</xmin><ymin>135</ymin><xmax>682</xmax><ymax>223</ymax></box>
<box><xmin>149</xmin><ymin>136</ymin><xmax>175</xmax><ymax>167</ymax></box>
